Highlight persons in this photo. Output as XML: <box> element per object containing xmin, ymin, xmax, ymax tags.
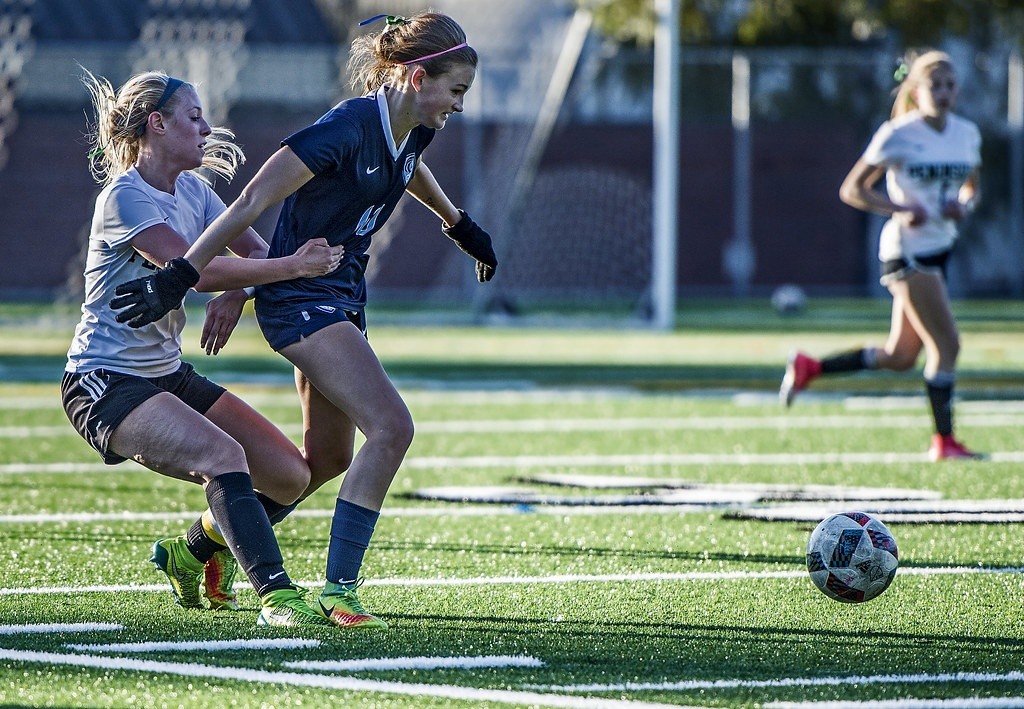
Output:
<box><xmin>109</xmin><ymin>10</ymin><xmax>497</xmax><ymax>623</ymax></box>
<box><xmin>781</xmin><ymin>49</ymin><xmax>990</xmax><ymax>462</ymax></box>
<box><xmin>61</xmin><ymin>64</ymin><xmax>344</xmax><ymax>628</ymax></box>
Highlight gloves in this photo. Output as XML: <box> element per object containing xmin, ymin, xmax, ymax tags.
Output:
<box><xmin>108</xmin><ymin>256</ymin><xmax>202</xmax><ymax>328</ymax></box>
<box><xmin>441</xmin><ymin>208</ymin><xmax>498</xmax><ymax>282</ymax></box>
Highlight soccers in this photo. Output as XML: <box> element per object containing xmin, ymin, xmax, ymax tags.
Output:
<box><xmin>805</xmin><ymin>510</ymin><xmax>899</xmax><ymax>604</ymax></box>
<box><xmin>770</xmin><ymin>282</ymin><xmax>809</xmax><ymax>319</ymax></box>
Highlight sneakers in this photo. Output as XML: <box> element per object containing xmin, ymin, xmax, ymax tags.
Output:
<box><xmin>148</xmin><ymin>536</ymin><xmax>204</xmax><ymax>610</ymax></box>
<box><xmin>256</xmin><ymin>583</ymin><xmax>334</xmax><ymax>628</ymax></box>
<box><xmin>779</xmin><ymin>352</ymin><xmax>813</xmax><ymax>411</ymax></box>
<box><xmin>202</xmin><ymin>548</ymin><xmax>238</xmax><ymax>611</ymax></box>
<box><xmin>932</xmin><ymin>436</ymin><xmax>984</xmax><ymax>462</ymax></box>
<box><xmin>315</xmin><ymin>576</ymin><xmax>388</xmax><ymax>629</ymax></box>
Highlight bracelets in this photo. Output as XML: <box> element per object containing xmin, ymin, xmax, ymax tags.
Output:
<box><xmin>243</xmin><ymin>286</ymin><xmax>255</xmax><ymax>299</ymax></box>
